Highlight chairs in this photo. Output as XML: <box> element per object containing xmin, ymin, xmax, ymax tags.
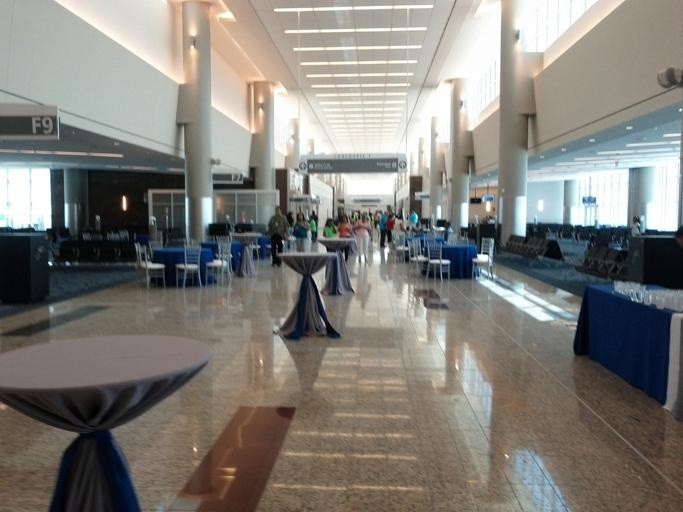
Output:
<box><xmin>132</xmin><ymin>235</ymin><xmax>232</xmax><ymax>289</ymax></box>
<box><xmin>501</xmin><ymin>221</ymin><xmax>631</xmax><ymax>283</ymax></box>
<box><xmin>406</xmin><ymin>228</ymin><xmax>495</xmax><ymax>284</ymax></box>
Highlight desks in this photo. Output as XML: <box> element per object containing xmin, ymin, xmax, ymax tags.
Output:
<box><xmin>581</xmin><ymin>281</ymin><xmax>682</xmax><ymax>419</ymax></box>
<box><xmin>198</xmin><ymin>240</ymin><xmax>242</xmax><ymax>270</ymax></box>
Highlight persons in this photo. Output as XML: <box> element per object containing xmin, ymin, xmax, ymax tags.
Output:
<box><xmin>675</xmin><ymin>227</ymin><xmax>683</xmax><ymax>248</ymax></box>
<box><xmin>268</xmin><ymin>205</ymin><xmax>453</xmax><ymax>268</ymax></box>
<box><xmin>630</xmin><ymin>216</ymin><xmax>642</xmax><ymax>236</ymax></box>
